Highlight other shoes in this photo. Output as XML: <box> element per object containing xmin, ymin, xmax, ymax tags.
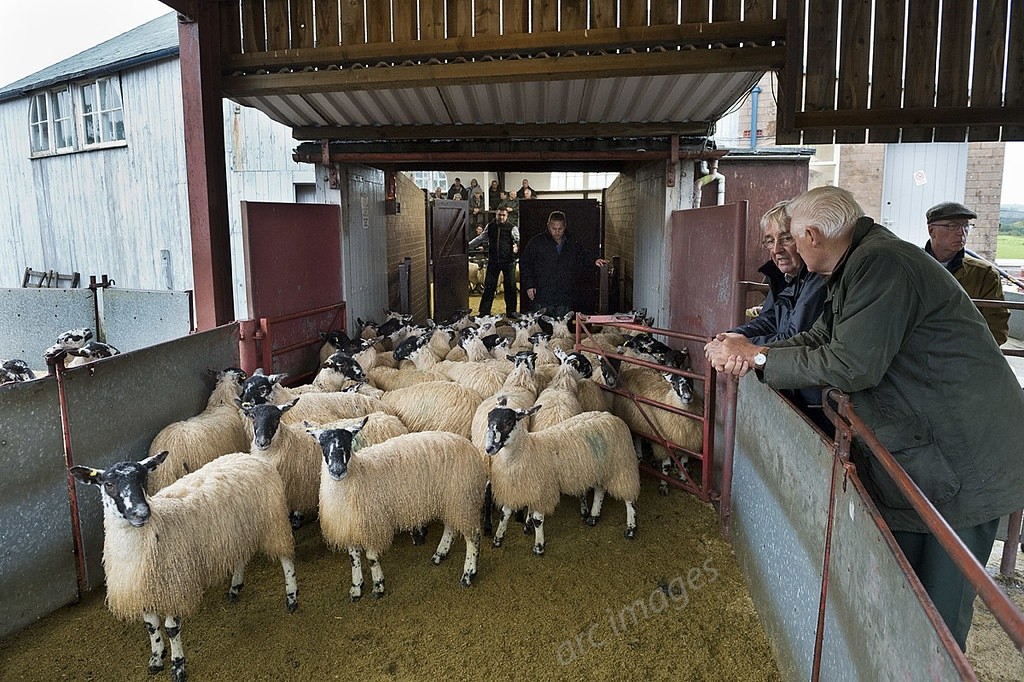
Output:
<box><xmin>507</xmin><ymin>311</ymin><xmax>520</xmax><ymax>320</ymax></box>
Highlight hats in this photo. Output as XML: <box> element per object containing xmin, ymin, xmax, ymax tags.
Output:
<box><xmin>927</xmin><ymin>201</ymin><xmax>979</xmax><ymax>226</ymax></box>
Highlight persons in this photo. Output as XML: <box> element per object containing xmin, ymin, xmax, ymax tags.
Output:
<box><xmin>433</xmin><ymin>177</ymin><xmax>537</xmax><ymax>267</ymax></box>
<box><xmin>704</xmin><ymin>187</ymin><xmax>1024</xmax><ymax>655</ymax></box>
<box><xmin>520</xmin><ymin>210</ymin><xmax>611</xmax><ymax>321</ymax></box>
<box><xmin>725</xmin><ymin>201</ymin><xmax>836</xmax><ymax>440</ymax></box>
<box><xmin>468</xmin><ymin>207</ymin><xmax>520</xmax><ymax>319</ymax></box>
<box><xmin>923</xmin><ymin>201</ymin><xmax>1010</xmax><ymax>346</ymax></box>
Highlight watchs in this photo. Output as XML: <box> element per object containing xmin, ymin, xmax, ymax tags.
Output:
<box><xmin>754</xmin><ymin>347</ymin><xmax>768</xmax><ymax>371</ymax></box>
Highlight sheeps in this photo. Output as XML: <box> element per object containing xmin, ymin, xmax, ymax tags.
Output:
<box><xmin>68</xmin><ymin>451</ymin><xmax>298</xmax><ymax>681</ymax></box>
<box><xmin>485</xmin><ymin>395</ymin><xmax>641</xmax><ymax>553</ymax></box>
<box><xmin>234</xmin><ymin>396</ymin><xmax>410</xmax><ymax>531</ymax></box>
<box><xmin>470</xmin><ymin>350</ymin><xmax>540</xmax><ymax>537</ymax></box>
<box><xmin>613</xmin><ymin>367</ymin><xmax>703</xmax><ymax>496</ymax></box>
<box><xmin>301</xmin><ymin>416</ymin><xmax>484</xmax><ymax>601</ymax></box>
<box><xmin>144</xmin><ymin>366</ymin><xmax>251</xmax><ymax>497</ymax></box>
<box><xmin>241</xmin><ymin>367</ymin><xmax>398</xmax><ymax>445</ymax></box>
<box><xmin>523</xmin><ymin>348</ymin><xmax>592</xmax><ymax>535</ymax></box>
<box><xmin>0</xmin><ymin>327</ymin><xmax>121</xmax><ymax>386</ymax></box>
<box><xmin>284</xmin><ymin>306</ymin><xmax>674</xmax><ymax>439</ymax></box>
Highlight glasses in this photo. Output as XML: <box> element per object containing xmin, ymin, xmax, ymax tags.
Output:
<box><xmin>764</xmin><ymin>236</ymin><xmax>794</xmax><ymax>250</ymax></box>
<box><xmin>933</xmin><ymin>222</ymin><xmax>975</xmax><ymax>232</ymax></box>
